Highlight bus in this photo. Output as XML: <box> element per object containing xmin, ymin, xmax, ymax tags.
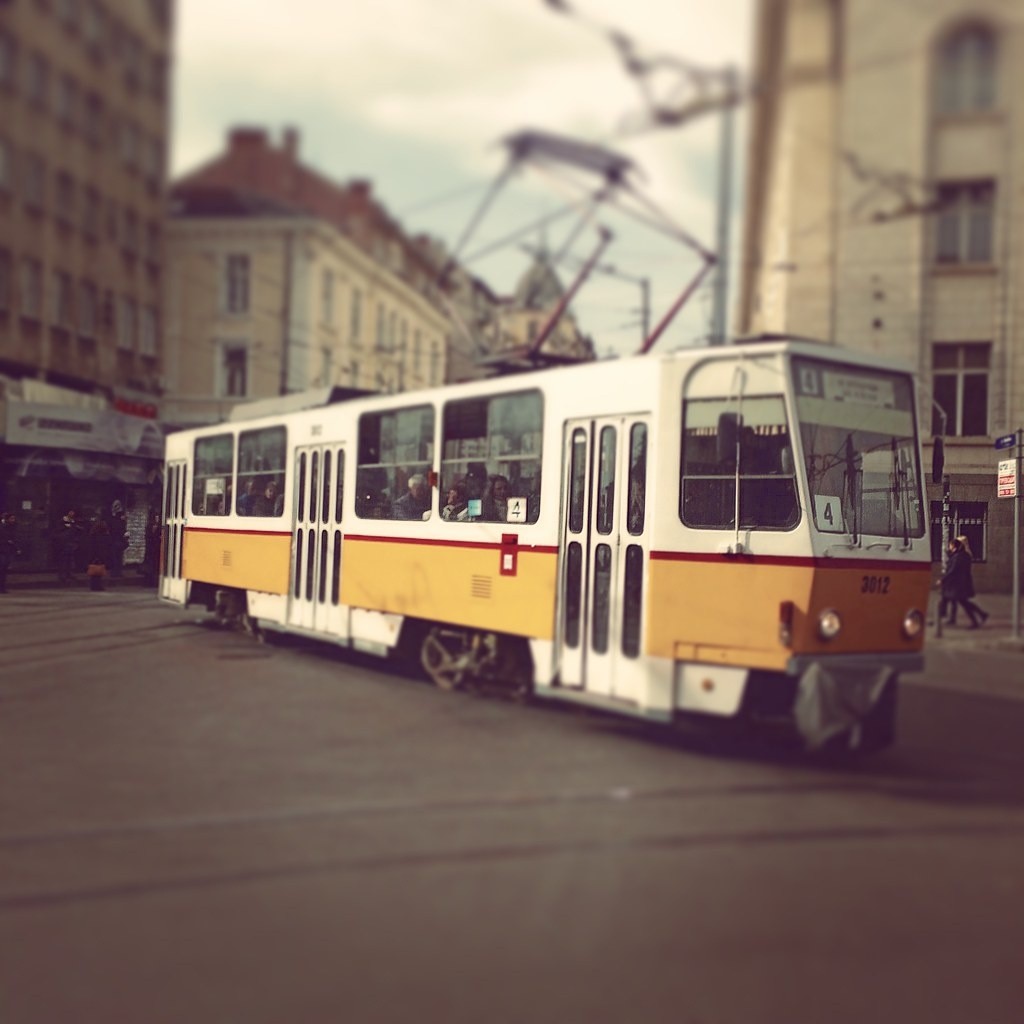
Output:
<box><xmin>157</xmin><ymin>334</ymin><xmax>949</xmax><ymax>746</ymax></box>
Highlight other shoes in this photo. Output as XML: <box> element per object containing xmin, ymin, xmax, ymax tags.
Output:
<box><xmin>982</xmin><ymin>613</ymin><xmax>991</xmax><ymax>625</ymax></box>
<box><xmin>944</xmin><ymin>619</ymin><xmax>958</xmax><ymax>628</ymax></box>
<box><xmin>969</xmin><ymin>623</ymin><xmax>980</xmax><ymax>631</ymax></box>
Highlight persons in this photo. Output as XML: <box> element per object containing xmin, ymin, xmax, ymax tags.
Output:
<box><xmin>0</xmin><ymin>514</ymin><xmax>21</xmax><ymax>594</ymax></box>
<box><xmin>442</xmin><ymin>476</ymin><xmax>512</xmax><ymax>523</ymax></box>
<box><xmin>56</xmin><ymin>506</ymin><xmax>109</xmax><ymax>590</ymax></box>
<box><xmin>193</xmin><ymin>456</ymin><xmax>280</xmax><ymax>517</ymax></box>
<box><xmin>113</xmin><ymin>511</ymin><xmax>129</xmax><ymax>578</ymax></box>
<box><xmin>356</xmin><ymin>469</ymin><xmax>431</xmax><ymax>520</ymax></box>
<box><xmin>935</xmin><ymin>535</ymin><xmax>990</xmax><ymax>629</ymax></box>
